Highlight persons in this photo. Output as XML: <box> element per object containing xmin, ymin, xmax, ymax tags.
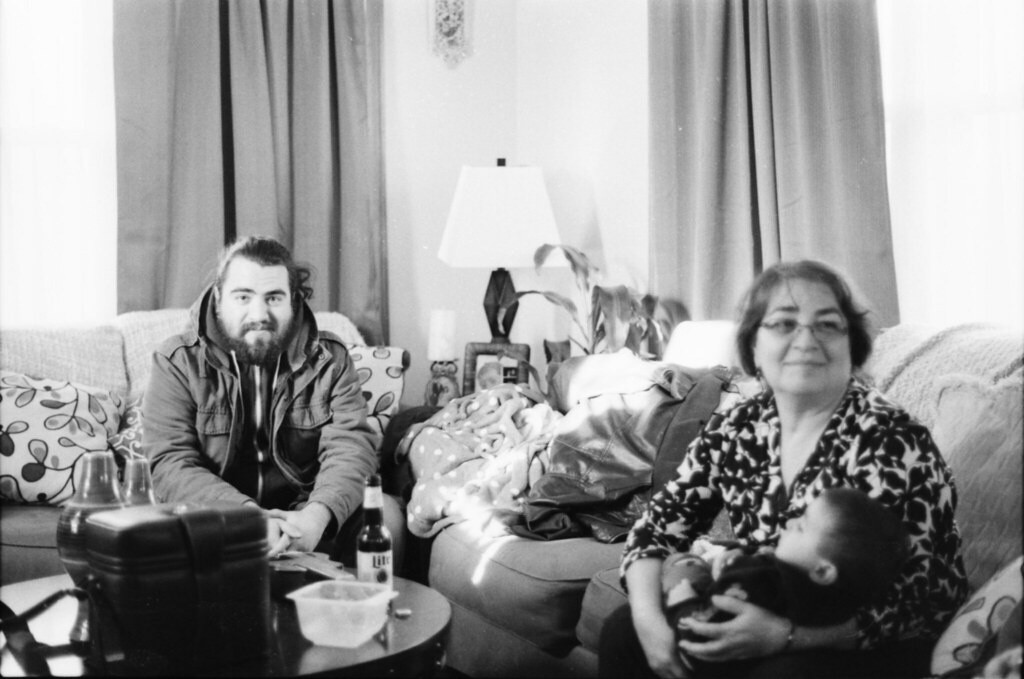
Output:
<box><xmin>593</xmin><ymin>255</ymin><xmax>973</xmax><ymax>679</ymax></box>
<box><xmin>621</xmin><ymin>483</ymin><xmax>908</xmax><ymax>668</ymax></box>
<box><xmin>137</xmin><ymin>235</ymin><xmax>379</xmax><ymax>567</ymax></box>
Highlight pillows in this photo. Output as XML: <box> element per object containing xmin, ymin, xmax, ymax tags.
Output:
<box><xmin>0</xmin><ymin>368</ymin><xmax>128</xmax><ymax>507</ymax></box>
<box><xmin>346</xmin><ymin>344</ymin><xmax>411</xmax><ymax>448</ymax></box>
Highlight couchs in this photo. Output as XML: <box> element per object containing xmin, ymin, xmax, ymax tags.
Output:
<box><xmin>0</xmin><ymin>309</ymin><xmax>365</xmax><ymax>587</ymax></box>
<box><xmin>425</xmin><ymin>320</ymin><xmax>1024</xmax><ymax>679</ymax></box>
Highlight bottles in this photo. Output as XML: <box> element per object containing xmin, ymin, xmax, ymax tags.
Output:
<box><xmin>56</xmin><ymin>449</ymin><xmax>122</xmax><ymax>643</ymax></box>
<box><xmin>120</xmin><ymin>458</ymin><xmax>155</xmax><ymax>507</ymax></box>
<box><xmin>356</xmin><ymin>473</ymin><xmax>392</xmax><ymax>591</ymax></box>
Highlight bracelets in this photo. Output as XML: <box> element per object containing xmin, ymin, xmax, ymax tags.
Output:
<box><xmin>780</xmin><ymin>620</ymin><xmax>796</xmax><ymax>655</ymax></box>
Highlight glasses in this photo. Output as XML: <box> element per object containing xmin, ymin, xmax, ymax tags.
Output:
<box><xmin>760</xmin><ymin>319</ymin><xmax>850</xmax><ymax>341</ymax></box>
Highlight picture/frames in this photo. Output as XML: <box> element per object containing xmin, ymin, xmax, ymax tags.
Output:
<box><xmin>462</xmin><ymin>342</ymin><xmax>531</xmax><ymax>396</ymax></box>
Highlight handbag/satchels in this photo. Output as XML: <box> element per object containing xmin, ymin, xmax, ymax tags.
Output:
<box><xmin>0</xmin><ymin>504</ymin><xmax>274</xmax><ymax>679</ymax></box>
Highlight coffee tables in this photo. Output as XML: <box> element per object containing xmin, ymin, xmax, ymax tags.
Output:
<box><xmin>0</xmin><ymin>570</ymin><xmax>452</xmax><ymax>679</ymax></box>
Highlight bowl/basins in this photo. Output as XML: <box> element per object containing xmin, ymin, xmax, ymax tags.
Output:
<box><xmin>284</xmin><ymin>580</ymin><xmax>399</xmax><ymax>648</ymax></box>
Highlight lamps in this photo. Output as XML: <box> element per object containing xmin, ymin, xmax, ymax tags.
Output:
<box><xmin>436</xmin><ymin>157</ymin><xmax>568</xmax><ymax>344</ymax></box>
